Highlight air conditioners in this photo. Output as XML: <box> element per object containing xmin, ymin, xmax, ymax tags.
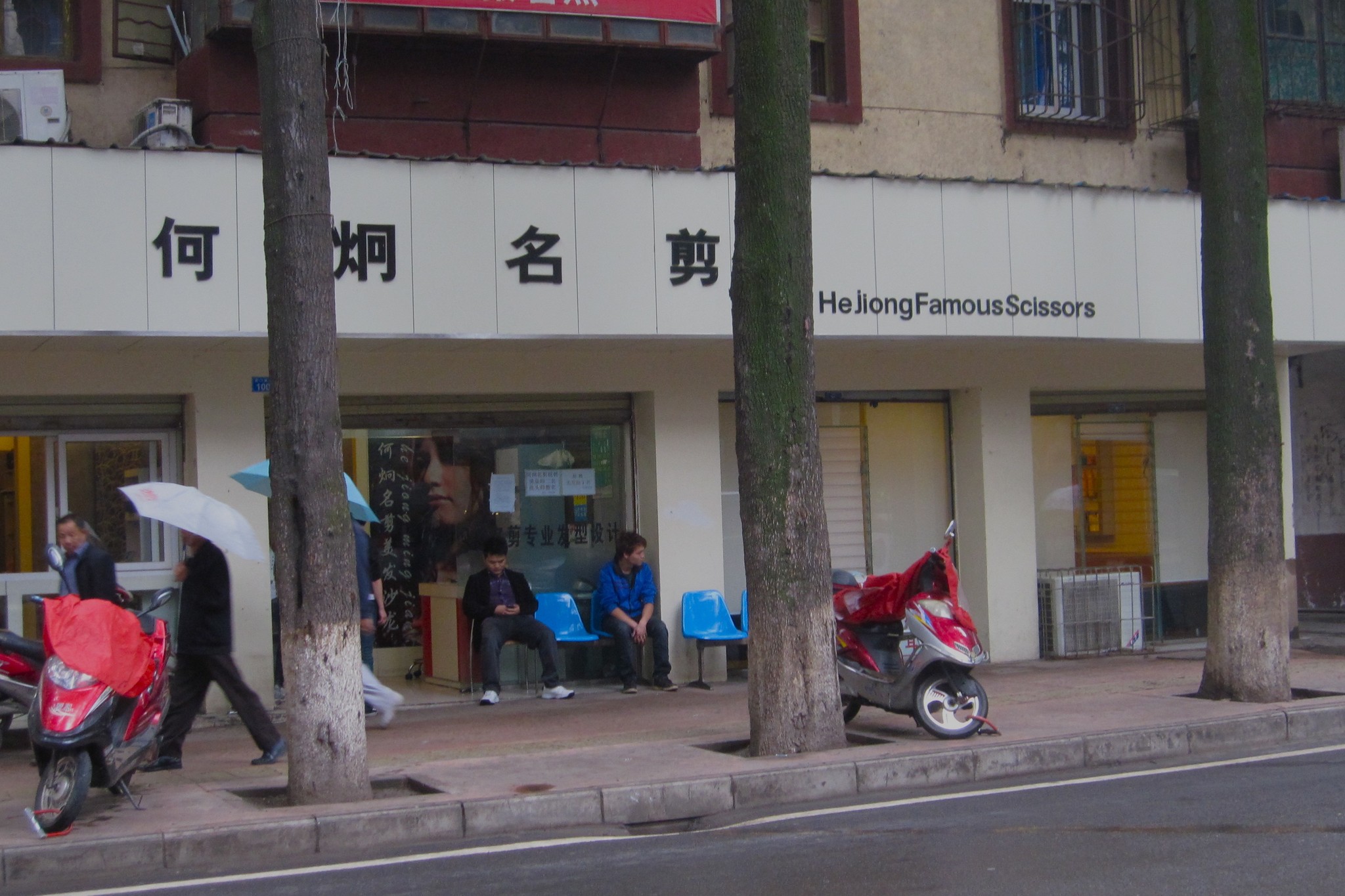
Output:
<box><xmin>0</xmin><ymin>69</ymin><xmax>69</xmax><ymax>143</ymax></box>
<box><xmin>132</xmin><ymin>102</ymin><xmax>193</xmax><ymax>149</ymax></box>
<box><xmin>1038</xmin><ymin>572</ymin><xmax>1143</xmax><ymax>657</ymax></box>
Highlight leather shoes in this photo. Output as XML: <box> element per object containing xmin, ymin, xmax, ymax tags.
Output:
<box><xmin>137</xmin><ymin>757</ymin><xmax>183</xmax><ymax>771</ymax></box>
<box><xmin>252</xmin><ymin>739</ymin><xmax>288</xmax><ymax>764</ymax></box>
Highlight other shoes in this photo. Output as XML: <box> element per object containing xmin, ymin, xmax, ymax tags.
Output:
<box><xmin>364</xmin><ymin>703</ymin><xmax>378</xmax><ymax>717</ymax></box>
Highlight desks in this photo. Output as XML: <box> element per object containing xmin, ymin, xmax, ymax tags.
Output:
<box><xmin>418</xmin><ymin>583</ymin><xmax>471</xmax><ymax>690</ymax></box>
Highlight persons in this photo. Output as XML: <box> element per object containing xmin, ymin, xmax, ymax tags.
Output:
<box><xmin>411</xmin><ymin>436</ymin><xmax>495</xmax><ymax>584</ymax></box>
<box><xmin>352</xmin><ymin>517</ymin><xmax>404</xmax><ymax>727</ymax></box>
<box><xmin>57</xmin><ymin>513</ymin><xmax>117</xmax><ymax>601</ymax></box>
<box><xmin>462</xmin><ymin>536</ymin><xmax>575</xmax><ymax>705</ymax></box>
<box><xmin>597</xmin><ymin>532</ymin><xmax>678</xmax><ymax>694</ymax></box>
<box><xmin>137</xmin><ymin>529</ymin><xmax>285</xmax><ymax>773</ymax></box>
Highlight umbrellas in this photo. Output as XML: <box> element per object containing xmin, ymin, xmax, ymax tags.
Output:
<box><xmin>117</xmin><ymin>482</ymin><xmax>265</xmax><ymax>560</ymax></box>
<box><xmin>228</xmin><ymin>459</ymin><xmax>380</xmax><ymax>523</ymax></box>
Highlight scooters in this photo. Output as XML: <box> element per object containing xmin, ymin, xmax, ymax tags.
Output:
<box><xmin>1</xmin><ymin>545</ymin><xmax>176</xmax><ymax>833</ymax></box>
<box><xmin>833</xmin><ymin>519</ymin><xmax>991</xmax><ymax>738</ymax></box>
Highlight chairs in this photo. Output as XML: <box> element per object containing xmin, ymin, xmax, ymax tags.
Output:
<box><xmin>535</xmin><ymin>589</ymin><xmax>749</xmax><ymax>691</ymax></box>
<box><xmin>504</xmin><ymin>640</ymin><xmax>529</xmax><ymax>695</ymax></box>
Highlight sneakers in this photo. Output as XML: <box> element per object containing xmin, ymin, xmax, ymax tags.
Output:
<box><xmin>622</xmin><ymin>680</ymin><xmax>636</xmax><ymax>693</ymax></box>
<box><xmin>654</xmin><ymin>675</ymin><xmax>678</xmax><ymax>690</ymax></box>
<box><xmin>542</xmin><ymin>685</ymin><xmax>575</xmax><ymax>700</ymax></box>
<box><xmin>479</xmin><ymin>690</ymin><xmax>499</xmax><ymax>705</ymax></box>
